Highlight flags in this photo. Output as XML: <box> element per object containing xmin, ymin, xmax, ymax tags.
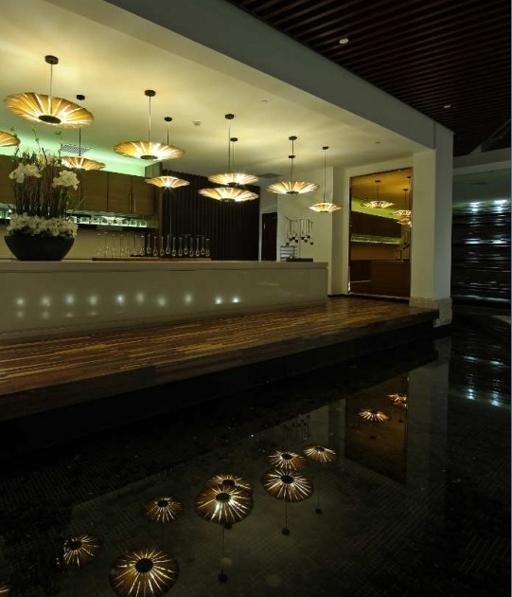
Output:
<box><xmin>4</xmin><ymin>225</ymin><xmax>75</xmax><ymax>260</ymax></box>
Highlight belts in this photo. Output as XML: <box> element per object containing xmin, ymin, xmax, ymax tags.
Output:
<box><xmin>95</xmin><ymin>230</ymin><xmax>211</xmax><ymax>258</ymax></box>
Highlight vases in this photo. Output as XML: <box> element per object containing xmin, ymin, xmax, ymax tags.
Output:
<box><xmin>4</xmin><ymin>124</ymin><xmax>81</xmax><ymax>240</ymax></box>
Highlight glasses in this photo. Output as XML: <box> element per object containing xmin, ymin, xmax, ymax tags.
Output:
<box><xmin>0</xmin><ymin>53</ymin><xmax>106</xmax><ymax>171</ymax></box>
<box><xmin>114</xmin><ymin>89</ymin><xmax>343</xmax><ymax>213</ymax></box>
